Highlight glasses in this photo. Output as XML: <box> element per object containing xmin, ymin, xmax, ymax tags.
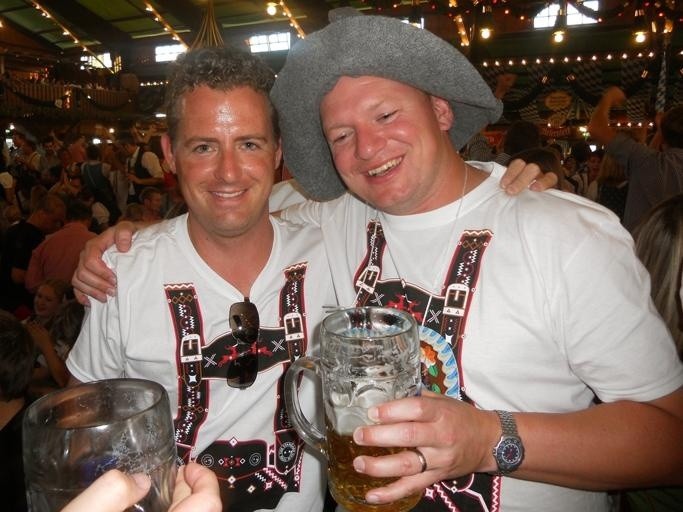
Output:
<box><xmin>226</xmin><ymin>297</ymin><xmax>259</xmax><ymax>389</ymax></box>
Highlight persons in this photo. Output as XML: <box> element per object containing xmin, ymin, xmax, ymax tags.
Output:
<box><xmin>58</xmin><ymin>460</ymin><xmax>225</xmax><ymax>511</ymax></box>
<box><xmin>1</xmin><ymin>316</ymin><xmax>49</xmax><ymax>511</ymax></box>
<box><xmin>458</xmin><ymin>84</ymin><xmax>682</xmax><ymax>228</ymax></box>
<box><xmin>590</xmin><ymin>198</ymin><xmax>683</xmax><ymax>512</ymax></box>
<box><xmin>58</xmin><ymin>45</ymin><xmax>560</xmax><ymax>511</ymax></box>
<box><xmin>70</xmin><ymin>12</ymin><xmax>682</xmax><ymax>511</ymax></box>
<box><xmin>0</xmin><ymin>126</ymin><xmax>191</xmax><ymax>397</ymax></box>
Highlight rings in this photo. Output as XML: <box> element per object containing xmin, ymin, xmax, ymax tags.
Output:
<box><xmin>411</xmin><ymin>449</ymin><xmax>427</xmax><ymax>473</ymax></box>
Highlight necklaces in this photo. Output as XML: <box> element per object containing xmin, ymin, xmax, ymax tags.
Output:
<box><xmin>376</xmin><ymin>159</ymin><xmax>468</xmax><ymax>326</ymax></box>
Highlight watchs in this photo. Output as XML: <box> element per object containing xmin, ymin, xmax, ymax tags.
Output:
<box><xmin>494</xmin><ymin>411</ymin><xmax>526</xmax><ymax>476</ymax></box>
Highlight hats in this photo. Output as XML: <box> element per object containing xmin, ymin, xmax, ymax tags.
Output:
<box><xmin>268</xmin><ymin>8</ymin><xmax>504</xmax><ymax>203</ymax></box>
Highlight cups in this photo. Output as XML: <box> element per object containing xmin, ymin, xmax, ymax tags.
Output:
<box><xmin>283</xmin><ymin>306</ymin><xmax>427</xmax><ymax>511</ymax></box>
<box><xmin>21</xmin><ymin>378</ymin><xmax>175</xmax><ymax>512</ymax></box>
